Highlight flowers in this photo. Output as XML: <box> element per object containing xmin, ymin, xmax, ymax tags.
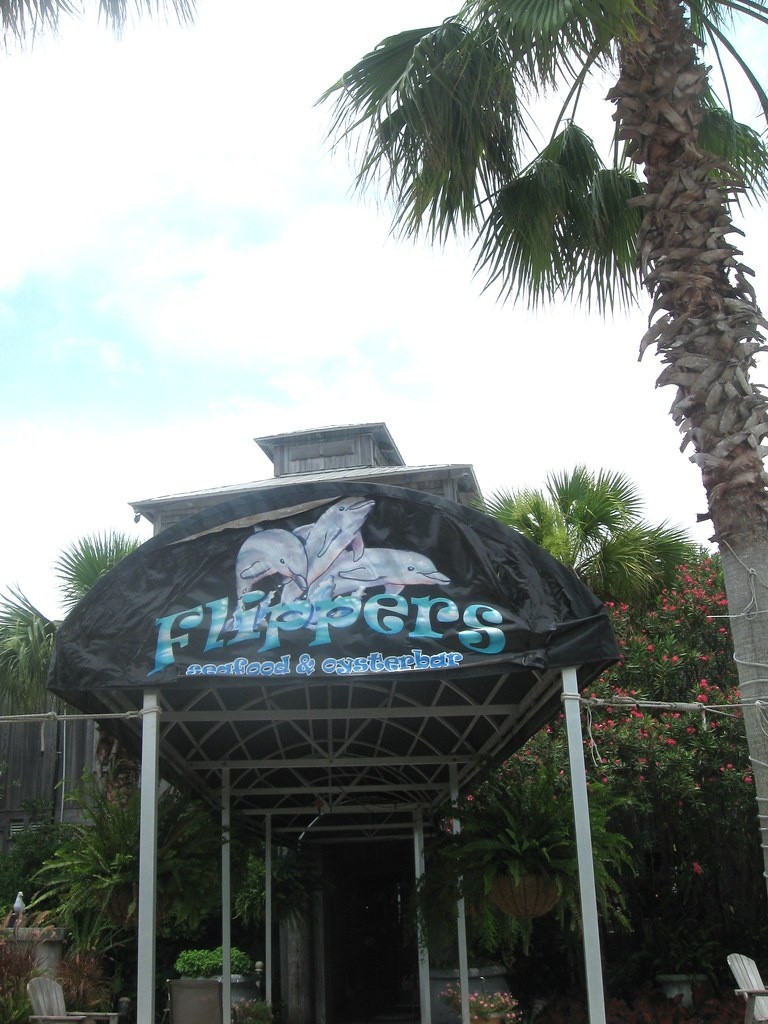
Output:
<box><xmin>438</xmin><ymin>982</ymin><xmax>523</xmax><ymax>1023</ymax></box>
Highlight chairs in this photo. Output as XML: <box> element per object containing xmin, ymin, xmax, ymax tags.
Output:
<box><xmin>26</xmin><ymin>976</ymin><xmax>122</xmax><ymax>1024</ymax></box>
<box><xmin>727</xmin><ymin>953</ymin><xmax>768</xmax><ymax>1024</ymax></box>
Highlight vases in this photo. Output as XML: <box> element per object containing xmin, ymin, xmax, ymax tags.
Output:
<box><xmin>472</xmin><ymin>1015</ymin><xmax>503</xmax><ymax>1024</ymax></box>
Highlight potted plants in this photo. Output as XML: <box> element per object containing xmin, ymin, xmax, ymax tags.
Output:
<box><xmin>173</xmin><ymin>944</ymin><xmax>263</xmax><ymax>1024</ymax></box>
<box><xmin>402</xmin><ymin>763</ymin><xmax>722</xmax><ymax>1024</ymax></box>
<box><xmin>23</xmin><ymin>763</ymin><xmax>336</xmax><ymax>933</ymax></box>
